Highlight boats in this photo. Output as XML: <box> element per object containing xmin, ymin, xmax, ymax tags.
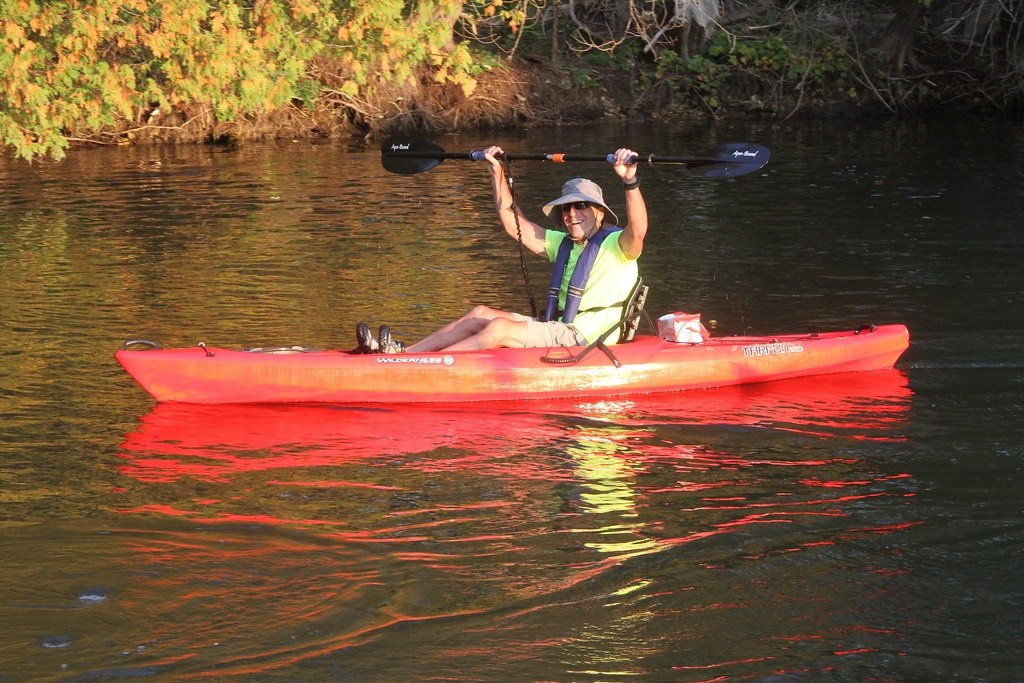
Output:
<box><xmin>115</xmin><ymin>320</ymin><xmax>909</xmax><ymax>406</ymax></box>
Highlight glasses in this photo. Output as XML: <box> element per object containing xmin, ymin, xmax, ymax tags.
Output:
<box><xmin>562</xmin><ymin>201</ymin><xmax>590</xmax><ymax>212</ymax></box>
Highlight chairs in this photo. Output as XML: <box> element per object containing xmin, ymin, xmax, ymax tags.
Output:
<box><xmin>620</xmin><ymin>276</ymin><xmax>649</xmax><ymax>342</ymax></box>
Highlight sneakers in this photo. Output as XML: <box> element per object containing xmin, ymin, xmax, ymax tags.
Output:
<box><xmin>378</xmin><ymin>324</ymin><xmax>405</xmax><ymax>353</ymax></box>
<box><xmin>355</xmin><ymin>322</ymin><xmax>378</xmax><ymax>352</ymax></box>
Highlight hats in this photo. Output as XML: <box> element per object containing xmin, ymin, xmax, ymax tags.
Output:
<box><xmin>542</xmin><ymin>177</ymin><xmax>619</xmax><ymax>227</ymax></box>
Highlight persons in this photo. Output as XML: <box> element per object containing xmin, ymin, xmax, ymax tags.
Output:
<box><xmin>356</xmin><ymin>146</ymin><xmax>647</xmax><ymax>355</ymax></box>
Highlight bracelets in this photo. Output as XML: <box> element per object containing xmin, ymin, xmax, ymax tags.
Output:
<box><xmin>624</xmin><ymin>178</ymin><xmax>641</xmax><ymax>190</ymax></box>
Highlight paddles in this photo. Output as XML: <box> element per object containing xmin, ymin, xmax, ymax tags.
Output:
<box><xmin>377</xmin><ymin>136</ymin><xmax>773</xmax><ymax>180</ymax></box>
